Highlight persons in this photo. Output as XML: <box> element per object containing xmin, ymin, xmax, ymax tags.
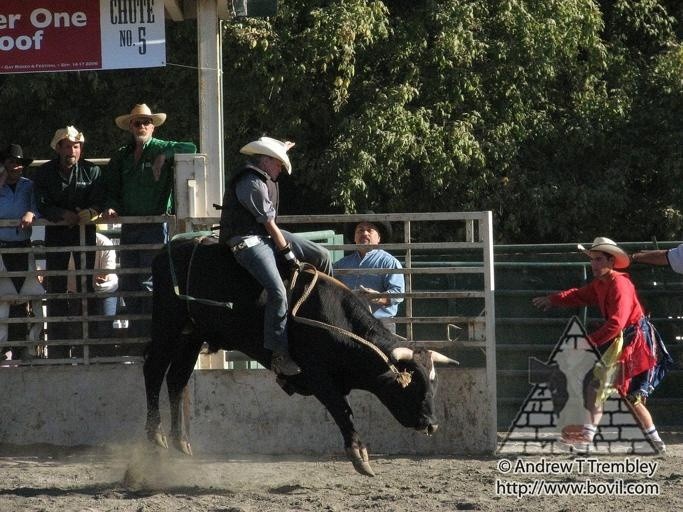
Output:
<box><xmin>101</xmin><ymin>102</ymin><xmax>198</xmax><ymax>355</ymax></box>
<box><xmin>218</xmin><ymin>135</ymin><xmax>334</xmax><ymax>376</ymax></box>
<box><xmin>532</xmin><ymin>235</ymin><xmax>670</xmax><ymax>459</ymax></box>
<box><xmin>0</xmin><ymin>142</ymin><xmax>41</xmax><ymax>364</ymax></box>
<box><xmin>36</xmin><ymin>125</ymin><xmax>105</xmax><ymax>359</ymax></box>
<box><xmin>331</xmin><ymin>210</ymin><xmax>405</xmax><ymax>334</ymax></box>
<box><xmin>628</xmin><ymin>242</ymin><xmax>683</xmax><ymax>276</ymax></box>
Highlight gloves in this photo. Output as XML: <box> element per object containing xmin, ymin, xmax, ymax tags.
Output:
<box><xmin>62</xmin><ymin>211</ymin><xmax>79</xmax><ymax>228</ymax></box>
<box><xmin>75</xmin><ymin>207</ymin><xmax>98</xmax><ymax>222</ymax></box>
<box><xmin>288</xmin><ymin>256</ymin><xmax>304</xmax><ymax>273</ymax></box>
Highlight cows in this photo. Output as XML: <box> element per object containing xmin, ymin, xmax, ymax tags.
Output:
<box><xmin>142</xmin><ymin>233</ymin><xmax>460</xmax><ymax>479</ymax></box>
<box><xmin>526</xmin><ymin>347</ymin><xmax>634</xmax><ymax>446</ymax></box>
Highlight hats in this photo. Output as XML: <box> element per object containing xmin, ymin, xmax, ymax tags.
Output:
<box><xmin>50</xmin><ymin>125</ymin><xmax>85</xmax><ymax>148</ymax></box>
<box><xmin>577</xmin><ymin>236</ymin><xmax>630</xmax><ymax>269</ymax></box>
<box><xmin>344</xmin><ymin>210</ymin><xmax>392</xmax><ymax>245</ymax></box>
<box><xmin>239</xmin><ymin>136</ymin><xmax>292</xmax><ymax>176</ymax></box>
<box><xmin>0</xmin><ymin>144</ymin><xmax>33</xmax><ymax>168</ymax></box>
<box><xmin>115</xmin><ymin>104</ymin><xmax>167</xmax><ymax>130</ymax></box>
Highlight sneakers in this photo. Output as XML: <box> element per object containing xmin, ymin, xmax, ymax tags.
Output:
<box><xmin>556</xmin><ymin>433</ymin><xmax>594</xmax><ymax>454</ymax></box>
<box><xmin>627</xmin><ymin>440</ymin><xmax>666</xmax><ymax>458</ymax></box>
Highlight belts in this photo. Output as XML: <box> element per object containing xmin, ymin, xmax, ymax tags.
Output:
<box><xmin>231</xmin><ymin>235</ymin><xmax>270</xmax><ymax>253</ymax></box>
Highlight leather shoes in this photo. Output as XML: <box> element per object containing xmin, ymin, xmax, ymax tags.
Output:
<box><xmin>271</xmin><ymin>353</ymin><xmax>301</xmax><ymax>377</ymax></box>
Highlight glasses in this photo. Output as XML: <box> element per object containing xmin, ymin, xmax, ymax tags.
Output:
<box><xmin>132</xmin><ymin>120</ymin><xmax>151</xmax><ymax>128</ymax></box>
